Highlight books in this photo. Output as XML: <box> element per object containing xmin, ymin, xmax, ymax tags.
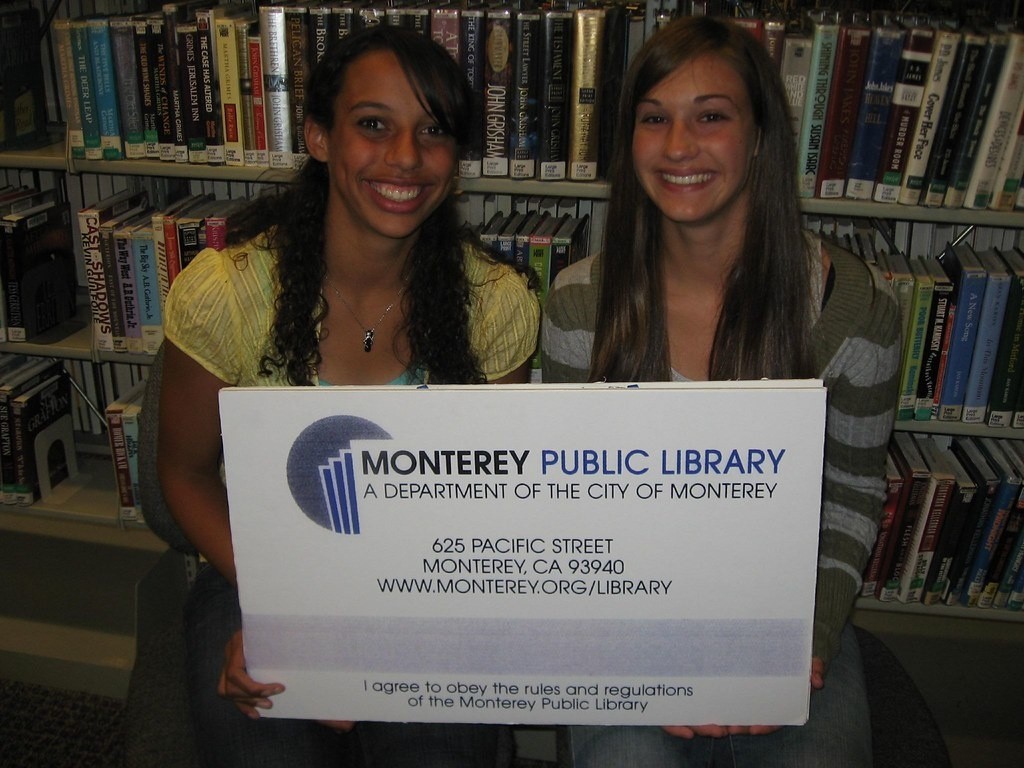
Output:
<box><xmin>0</xmin><ymin>0</ymin><xmax>1024</xmax><ymax>612</ymax></box>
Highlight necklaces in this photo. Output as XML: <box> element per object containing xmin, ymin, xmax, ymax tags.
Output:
<box><xmin>323</xmin><ymin>258</ymin><xmax>420</xmax><ymax>353</ymax></box>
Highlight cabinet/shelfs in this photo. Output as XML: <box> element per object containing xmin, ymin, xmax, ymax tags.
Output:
<box><xmin>0</xmin><ymin>0</ymin><xmax>1024</xmax><ymax>768</ymax></box>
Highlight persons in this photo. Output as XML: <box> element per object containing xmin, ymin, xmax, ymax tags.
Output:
<box><xmin>157</xmin><ymin>24</ymin><xmax>541</xmax><ymax>767</ymax></box>
<box><xmin>540</xmin><ymin>18</ymin><xmax>903</xmax><ymax>768</ymax></box>
